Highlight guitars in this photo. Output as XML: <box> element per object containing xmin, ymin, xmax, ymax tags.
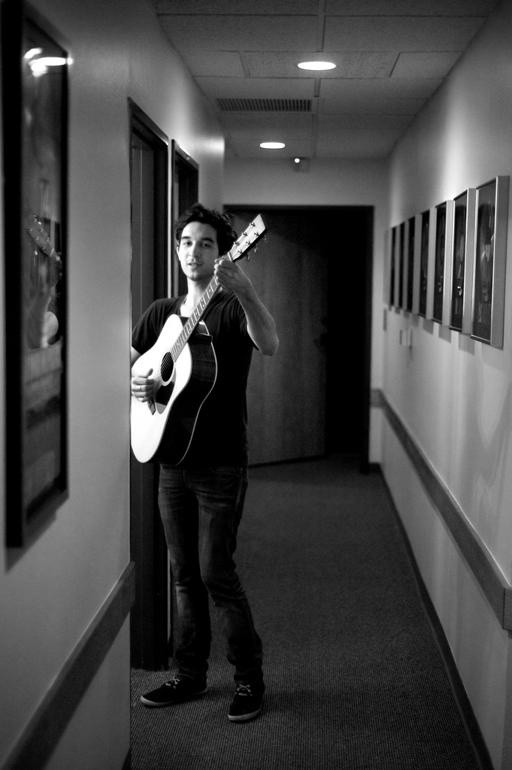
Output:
<box><xmin>130</xmin><ymin>215</ymin><xmax>267</xmax><ymax>464</ymax></box>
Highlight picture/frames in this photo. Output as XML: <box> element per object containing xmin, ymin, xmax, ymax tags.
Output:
<box><xmin>4</xmin><ymin>2</ymin><xmax>73</xmax><ymax>552</ymax></box>
<box><xmin>389</xmin><ymin>176</ymin><xmax>508</xmax><ymax>350</ymax></box>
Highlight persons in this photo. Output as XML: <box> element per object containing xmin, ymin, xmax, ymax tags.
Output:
<box><xmin>129</xmin><ymin>201</ymin><xmax>283</xmax><ymax>725</ymax></box>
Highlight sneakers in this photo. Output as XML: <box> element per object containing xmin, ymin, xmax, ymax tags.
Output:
<box><xmin>227</xmin><ymin>674</ymin><xmax>267</xmax><ymax>721</ymax></box>
<box><xmin>140</xmin><ymin>673</ymin><xmax>208</xmax><ymax>706</ymax></box>
